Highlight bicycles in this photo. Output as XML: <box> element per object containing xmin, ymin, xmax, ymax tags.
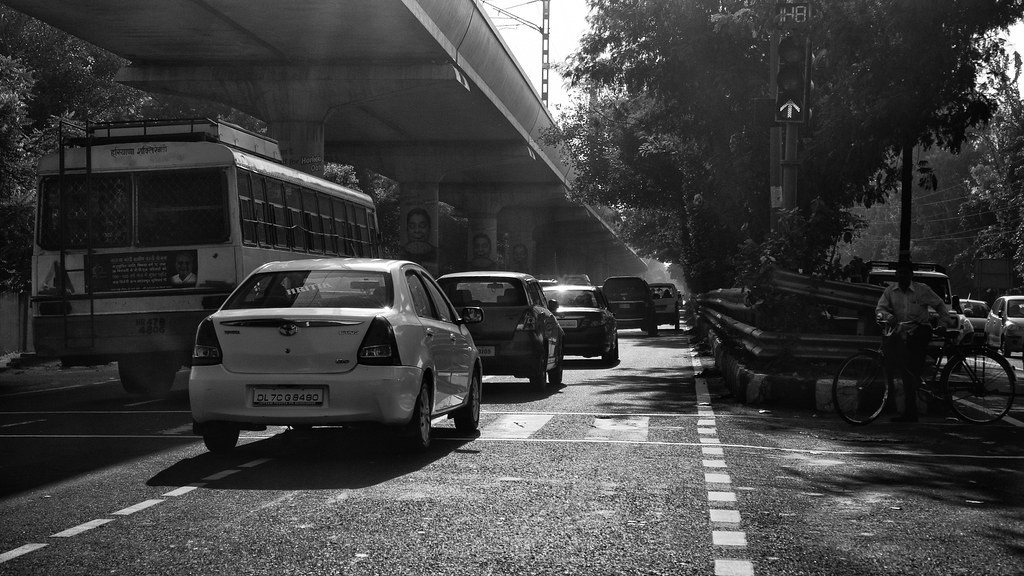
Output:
<box><xmin>831</xmin><ymin>311</ymin><xmax>1017</xmax><ymax>425</ymax></box>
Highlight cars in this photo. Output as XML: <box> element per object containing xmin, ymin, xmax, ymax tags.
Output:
<box><xmin>602</xmin><ymin>276</ymin><xmax>658</xmax><ymax>336</ymax></box>
<box><xmin>983</xmin><ymin>295</ymin><xmax>1024</xmax><ymax>357</ymax></box>
<box><xmin>542</xmin><ymin>286</ymin><xmax>618</xmax><ymax>364</ymax></box>
<box><xmin>436</xmin><ymin>271</ymin><xmax>564</xmax><ymax>392</ymax></box>
<box><xmin>958</xmin><ymin>294</ymin><xmax>991</xmax><ymax>337</ymax></box>
<box><xmin>188</xmin><ymin>259</ymin><xmax>482</xmax><ymax>454</ymax></box>
<box><xmin>647</xmin><ymin>282</ymin><xmax>681</xmax><ymax>329</ymax></box>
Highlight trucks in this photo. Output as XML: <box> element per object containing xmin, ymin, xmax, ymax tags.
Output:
<box><xmin>865</xmin><ymin>260</ymin><xmax>975</xmax><ymax>371</ymax></box>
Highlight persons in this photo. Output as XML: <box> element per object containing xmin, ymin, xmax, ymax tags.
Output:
<box><xmin>468</xmin><ymin>234</ymin><xmax>496</xmax><ymax>266</ymax></box>
<box><xmin>510</xmin><ymin>245</ymin><xmax>532</xmax><ymax>272</ymax></box>
<box><xmin>875</xmin><ymin>261</ymin><xmax>954</xmax><ymax>423</ymax></box>
<box><xmin>400</xmin><ymin>208</ymin><xmax>436</xmax><ymax>264</ymax></box>
<box><xmin>171</xmin><ymin>252</ymin><xmax>197</xmax><ymax>283</ymax></box>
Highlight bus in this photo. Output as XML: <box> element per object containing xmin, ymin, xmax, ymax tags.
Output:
<box><xmin>29</xmin><ymin>113</ymin><xmax>384</xmax><ymax>398</ymax></box>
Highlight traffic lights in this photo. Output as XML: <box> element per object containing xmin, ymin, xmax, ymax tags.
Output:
<box><xmin>774</xmin><ymin>34</ymin><xmax>810</xmax><ymax>123</ymax></box>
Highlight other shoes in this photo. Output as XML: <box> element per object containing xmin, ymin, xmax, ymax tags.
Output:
<box><xmin>912</xmin><ymin>376</ymin><xmax>927</xmax><ymax>388</ymax></box>
<box><xmin>891</xmin><ymin>416</ymin><xmax>918</xmax><ymax>423</ymax></box>
